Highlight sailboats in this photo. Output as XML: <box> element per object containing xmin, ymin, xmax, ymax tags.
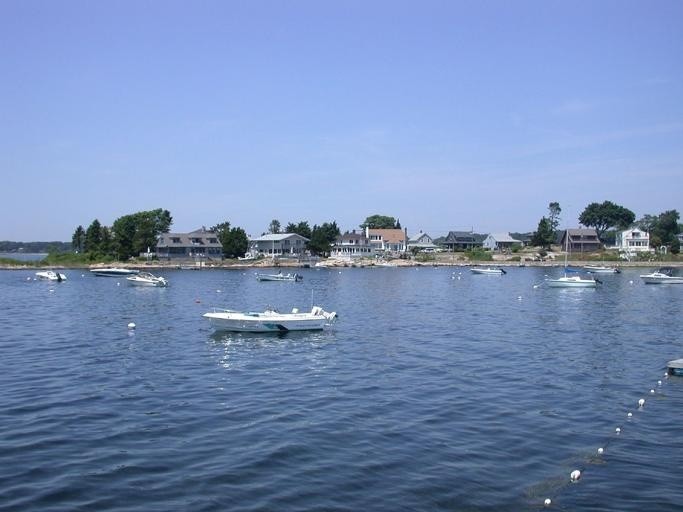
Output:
<box><xmin>543</xmin><ymin>230</ymin><xmax>598</xmax><ymax>288</ymax></box>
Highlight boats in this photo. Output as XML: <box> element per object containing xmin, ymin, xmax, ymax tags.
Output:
<box><xmin>200</xmin><ymin>305</ymin><xmax>337</xmax><ymax>333</ymax></box>
<box><xmin>583</xmin><ymin>263</ymin><xmax>622</xmax><ymax>274</ymax></box>
<box><xmin>640</xmin><ymin>266</ymin><xmax>682</xmax><ymax>284</ymax></box>
<box><xmin>469</xmin><ymin>266</ymin><xmax>506</xmax><ymax>275</ymax></box>
<box><xmin>34</xmin><ymin>270</ymin><xmax>66</xmax><ymax>283</ymax></box>
<box><xmin>252</xmin><ymin>263</ymin><xmax>303</xmax><ymax>283</ymax></box>
<box><xmin>126</xmin><ymin>271</ymin><xmax>168</xmax><ymax>288</ymax></box>
<box><xmin>89</xmin><ymin>263</ymin><xmax>139</xmax><ymax>278</ymax></box>
